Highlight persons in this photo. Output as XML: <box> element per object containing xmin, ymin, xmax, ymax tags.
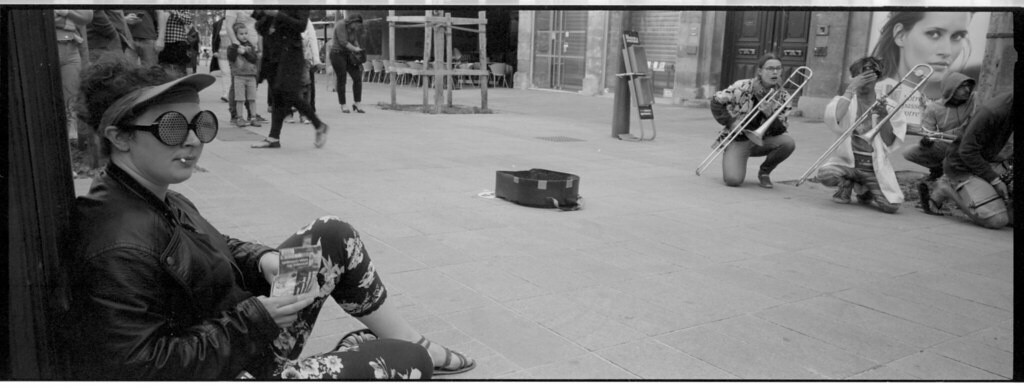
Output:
<box><xmin>58</xmin><ymin>58</ymin><xmax>476</xmax><ymax>379</ymax></box>
<box><xmin>903</xmin><ymin>71</ymin><xmax>977</xmax><ymax>180</ymax></box>
<box><xmin>252</xmin><ymin>10</ymin><xmax>330</xmax><ymax>148</ymax></box>
<box><xmin>53</xmin><ymin>9</ymin><xmax>134</xmax><ymax>176</ymax></box>
<box><xmin>123</xmin><ymin>8</ymin><xmax>165</xmax><ymax>66</ymax></box>
<box><xmin>227</xmin><ymin>24</ymin><xmax>263</xmax><ymax>127</ymax></box>
<box><xmin>225</xmin><ymin>10</ymin><xmax>268</xmax><ymax>126</ymax></box>
<box><xmin>711</xmin><ymin>53</ymin><xmax>796</xmax><ymax>188</ymax></box>
<box><xmin>874</xmin><ymin>11</ymin><xmax>974</xmax><ymax>124</ymax></box>
<box><xmin>917</xmin><ymin>90</ymin><xmax>1016</xmax><ymax>229</ymax></box>
<box><xmin>330</xmin><ymin>13</ymin><xmax>366</xmax><ymax>114</ymax></box>
<box><xmin>817</xmin><ymin>56</ymin><xmax>907</xmax><ymax>215</ymax></box>
<box><xmin>162</xmin><ymin>8</ymin><xmax>192</xmax><ymax>62</ymax></box>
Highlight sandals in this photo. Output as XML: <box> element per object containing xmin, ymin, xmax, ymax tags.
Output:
<box><xmin>416</xmin><ymin>336</ymin><xmax>476</xmax><ymax>375</ymax></box>
<box><xmin>331</xmin><ymin>328</ymin><xmax>378</xmax><ymax>352</ymax></box>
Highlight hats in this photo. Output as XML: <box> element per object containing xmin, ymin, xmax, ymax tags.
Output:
<box><xmin>96</xmin><ymin>72</ymin><xmax>217</xmax><ymax>137</ymax></box>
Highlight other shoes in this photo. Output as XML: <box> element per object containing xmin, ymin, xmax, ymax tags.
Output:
<box><xmin>832</xmin><ymin>185</ymin><xmax>853</xmax><ymax>203</ymax></box>
<box><xmin>284</xmin><ymin>115</ymin><xmax>294</xmax><ymax>123</ymax></box>
<box><xmin>317</xmin><ymin>123</ymin><xmax>329</xmax><ymax>148</ymax></box>
<box><xmin>247</xmin><ymin>114</ymin><xmax>265</xmax><ymax>121</ymax></box>
<box><xmin>857</xmin><ymin>192</ymin><xmax>872</xmax><ymax>204</ymax></box>
<box><xmin>231</xmin><ymin>117</ymin><xmax>237</xmax><ymax>123</ymax></box>
<box><xmin>926</xmin><ymin>167</ymin><xmax>944</xmax><ymax>180</ymax></box>
<box><xmin>236</xmin><ymin>118</ymin><xmax>246</xmax><ymax>127</ymax></box>
<box><xmin>302</xmin><ymin>115</ymin><xmax>311</xmax><ymax>124</ymax></box>
<box><xmin>250</xmin><ymin>119</ymin><xmax>262</xmax><ymax>127</ymax></box>
<box><xmin>758</xmin><ymin>171</ymin><xmax>774</xmax><ymax>188</ymax></box>
<box><xmin>251</xmin><ymin>139</ymin><xmax>281</xmax><ymax>148</ymax></box>
<box><xmin>220</xmin><ymin>96</ymin><xmax>230</xmax><ymax>102</ymax></box>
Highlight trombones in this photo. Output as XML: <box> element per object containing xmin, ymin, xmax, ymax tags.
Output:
<box><xmin>795</xmin><ymin>63</ymin><xmax>934</xmax><ymax>188</ymax></box>
<box><xmin>695</xmin><ymin>66</ymin><xmax>814</xmax><ymax>177</ymax></box>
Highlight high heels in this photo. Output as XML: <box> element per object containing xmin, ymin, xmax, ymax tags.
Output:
<box><xmin>352</xmin><ymin>103</ymin><xmax>365</xmax><ymax>114</ymax></box>
<box><xmin>341</xmin><ymin>105</ymin><xmax>350</xmax><ymax>113</ymax></box>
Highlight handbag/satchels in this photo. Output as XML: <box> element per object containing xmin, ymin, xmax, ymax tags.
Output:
<box><xmin>352</xmin><ymin>49</ymin><xmax>367</xmax><ymax>63</ymax></box>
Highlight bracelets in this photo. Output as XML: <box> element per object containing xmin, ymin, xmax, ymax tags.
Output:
<box><xmin>846</xmin><ymin>90</ymin><xmax>854</xmax><ymax>93</ymax></box>
<box><xmin>991</xmin><ymin>177</ymin><xmax>1002</xmax><ymax>185</ymax></box>
<box><xmin>841</xmin><ymin>96</ymin><xmax>851</xmax><ymax>102</ymax></box>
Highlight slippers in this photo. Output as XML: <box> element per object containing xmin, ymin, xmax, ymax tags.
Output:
<box><xmin>918</xmin><ymin>182</ymin><xmax>943</xmax><ymax>216</ymax></box>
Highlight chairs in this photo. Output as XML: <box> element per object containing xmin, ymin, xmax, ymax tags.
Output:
<box><xmin>360</xmin><ymin>58</ymin><xmax>513</xmax><ymax>89</ymax></box>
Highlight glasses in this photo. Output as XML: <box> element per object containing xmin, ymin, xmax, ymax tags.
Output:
<box><xmin>120</xmin><ymin>110</ymin><xmax>219</xmax><ymax>146</ymax></box>
<box><xmin>762</xmin><ymin>67</ymin><xmax>784</xmax><ymax>72</ymax></box>
<box><xmin>958</xmin><ymin>83</ymin><xmax>974</xmax><ymax>90</ymax></box>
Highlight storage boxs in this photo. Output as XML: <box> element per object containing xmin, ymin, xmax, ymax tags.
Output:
<box><xmin>496</xmin><ymin>169</ymin><xmax>583</xmax><ymax>211</ymax></box>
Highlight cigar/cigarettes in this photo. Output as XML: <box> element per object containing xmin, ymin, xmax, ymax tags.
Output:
<box><xmin>180</xmin><ymin>158</ymin><xmax>186</xmax><ymax>163</ymax></box>
